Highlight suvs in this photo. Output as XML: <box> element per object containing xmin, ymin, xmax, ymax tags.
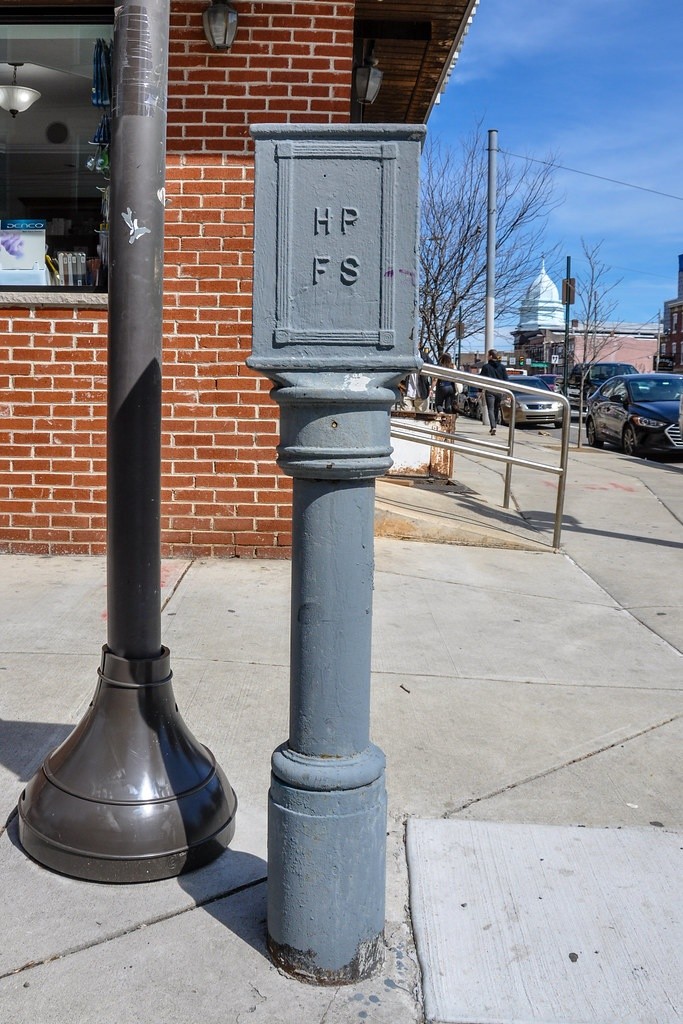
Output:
<box><xmin>560</xmin><ymin>361</ymin><xmax>640</xmax><ymax>410</ymax></box>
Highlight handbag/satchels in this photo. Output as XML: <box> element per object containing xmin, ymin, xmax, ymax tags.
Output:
<box><xmin>455</xmin><ymin>383</ymin><xmax>463</xmax><ymax>393</ymax></box>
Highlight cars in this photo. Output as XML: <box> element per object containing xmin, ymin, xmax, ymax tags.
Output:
<box><xmin>444</xmin><ymin>373</ymin><xmax>565</xmax><ymax>422</ymax></box>
<box><xmin>586</xmin><ymin>374</ymin><xmax>683</xmax><ymax>457</ymax></box>
<box><xmin>476</xmin><ymin>375</ymin><xmax>564</xmax><ymax>429</ymax></box>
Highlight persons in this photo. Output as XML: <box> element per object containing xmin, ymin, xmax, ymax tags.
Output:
<box><xmin>401</xmin><ymin>346</ymin><xmax>438</xmax><ymax>412</ymax></box>
<box><xmin>479</xmin><ymin>348</ymin><xmax>508</xmax><ymax>436</ymax></box>
<box><xmin>429</xmin><ymin>351</ymin><xmax>458</xmax><ymax>416</ymax></box>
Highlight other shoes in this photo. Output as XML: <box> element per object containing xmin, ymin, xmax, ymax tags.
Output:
<box><xmin>490</xmin><ymin>428</ymin><xmax>496</xmax><ymax>435</ymax></box>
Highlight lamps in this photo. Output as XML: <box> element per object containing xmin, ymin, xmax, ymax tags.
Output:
<box><xmin>0</xmin><ymin>62</ymin><xmax>43</xmax><ymax>121</ymax></box>
